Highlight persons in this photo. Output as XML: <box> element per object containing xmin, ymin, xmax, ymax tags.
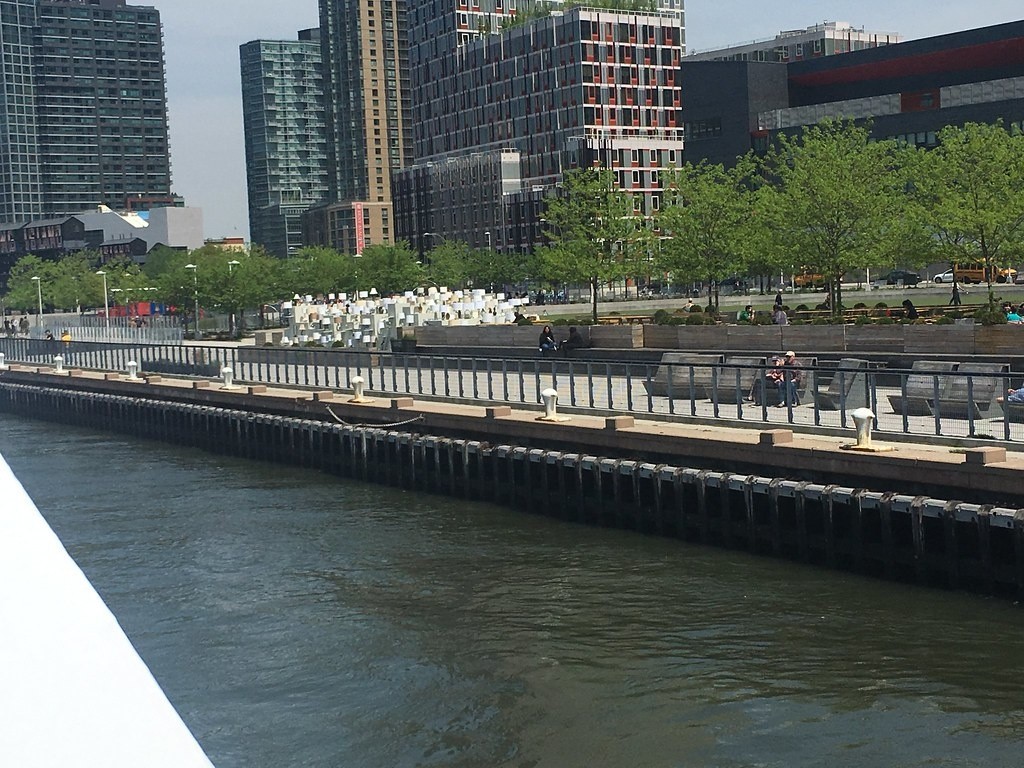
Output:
<box><xmin>902</xmin><ymin>299</ymin><xmax>918</xmax><ymax>319</ymax></box>
<box><xmin>682</xmin><ymin>300</ymin><xmax>694</xmax><ymax>312</ymax></box>
<box><xmin>775</xmin><ymin>289</ymin><xmax>786</xmax><ymax>306</ymax></box>
<box><xmin>61</xmin><ymin>330</ymin><xmax>71</xmax><ymax>345</ymax></box>
<box><xmin>997</xmin><ymin>387</ymin><xmax>1024</xmax><ymax>403</ymax></box>
<box><xmin>4</xmin><ymin>315</ymin><xmax>30</xmax><ymax>336</ymax></box>
<box><xmin>556</xmin><ymin>327</ymin><xmax>582</xmax><ymax>349</ymax></box>
<box><xmin>513</xmin><ymin>312</ymin><xmax>533</xmax><ymax>326</ymax></box>
<box><xmin>43</xmin><ymin>330</ymin><xmax>53</xmax><ymax>340</ymax></box>
<box><xmin>129</xmin><ymin>316</ymin><xmax>147</xmax><ymax>327</ymax></box>
<box><xmin>742</xmin><ymin>351</ymin><xmax>802</xmax><ymax>408</ymax></box>
<box><xmin>949</xmin><ymin>279</ymin><xmax>964</xmax><ymax>305</ymax></box>
<box><xmin>1004</xmin><ymin>301</ymin><xmax>1024</xmax><ymax>324</ymax></box>
<box><xmin>773</xmin><ymin>304</ymin><xmax>788</xmax><ymax>324</ymax></box>
<box><xmin>539</xmin><ymin>325</ymin><xmax>557</xmax><ymax>351</ymax></box>
<box><xmin>739</xmin><ymin>305</ymin><xmax>755</xmax><ymax>321</ymax></box>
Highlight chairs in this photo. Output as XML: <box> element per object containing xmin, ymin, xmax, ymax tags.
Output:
<box><xmin>527</xmin><ymin>315</ymin><xmax>536</xmax><ymax>320</ymax></box>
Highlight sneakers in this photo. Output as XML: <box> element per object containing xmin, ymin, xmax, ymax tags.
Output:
<box><xmin>751</xmin><ymin>403</ymin><xmax>760</xmax><ymax>407</ymax></box>
<box><xmin>742</xmin><ymin>397</ymin><xmax>754</xmax><ymax>402</ymax></box>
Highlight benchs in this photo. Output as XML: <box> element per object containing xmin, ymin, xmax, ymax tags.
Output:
<box><xmin>642</xmin><ymin>350</ymin><xmax>1009</xmax><ymax>417</ymax></box>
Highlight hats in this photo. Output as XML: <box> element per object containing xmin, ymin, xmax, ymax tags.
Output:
<box><xmin>784</xmin><ymin>350</ymin><xmax>795</xmax><ymax>357</ymax></box>
<box><xmin>772</xmin><ymin>355</ymin><xmax>780</xmax><ymax>362</ymax></box>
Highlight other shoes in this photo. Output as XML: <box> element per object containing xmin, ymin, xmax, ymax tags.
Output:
<box><xmin>777</xmin><ymin>403</ymin><xmax>785</xmax><ymax>408</ymax></box>
<box><xmin>791</xmin><ymin>403</ymin><xmax>798</xmax><ymax>407</ymax></box>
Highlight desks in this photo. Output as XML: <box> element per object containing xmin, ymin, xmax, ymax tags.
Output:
<box><xmin>597</xmin><ymin>315</ymin><xmax>653</xmax><ymax>325</ymax></box>
<box><xmin>532</xmin><ymin>320</ymin><xmax>550</xmax><ymax>324</ymax></box>
<box><xmin>796</xmin><ymin>308</ymin><xmax>980</xmax><ymax>323</ymax></box>
